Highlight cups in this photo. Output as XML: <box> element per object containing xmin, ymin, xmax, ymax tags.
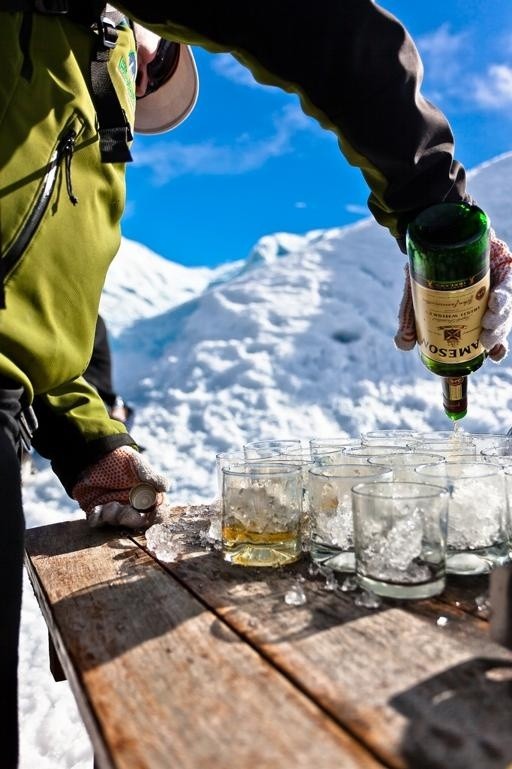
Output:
<box><xmin>215</xmin><ymin>430</ymin><xmax>511</xmax><ymax>600</ymax></box>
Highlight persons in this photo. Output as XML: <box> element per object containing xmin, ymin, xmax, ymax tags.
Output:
<box><xmin>85</xmin><ymin>313</ymin><xmax>137</xmax><ymax>433</ymax></box>
<box><xmin>1</xmin><ymin>1</ymin><xmax>510</xmax><ymax>769</ymax></box>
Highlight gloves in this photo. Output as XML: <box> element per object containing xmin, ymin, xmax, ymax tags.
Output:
<box><xmin>393</xmin><ymin>227</ymin><xmax>511</xmax><ymax>362</ymax></box>
<box><xmin>70</xmin><ymin>445</ymin><xmax>172</xmax><ymax>529</ymax></box>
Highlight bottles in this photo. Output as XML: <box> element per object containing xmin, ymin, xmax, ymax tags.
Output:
<box><xmin>404</xmin><ymin>198</ymin><xmax>491</xmax><ymax>422</ymax></box>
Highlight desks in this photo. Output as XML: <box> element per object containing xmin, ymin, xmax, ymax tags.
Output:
<box><xmin>23</xmin><ymin>504</ymin><xmax>512</xmax><ymax>768</ymax></box>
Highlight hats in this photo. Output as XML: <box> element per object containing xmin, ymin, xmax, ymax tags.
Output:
<box><xmin>134</xmin><ymin>43</ymin><xmax>200</xmax><ymax>134</ymax></box>
